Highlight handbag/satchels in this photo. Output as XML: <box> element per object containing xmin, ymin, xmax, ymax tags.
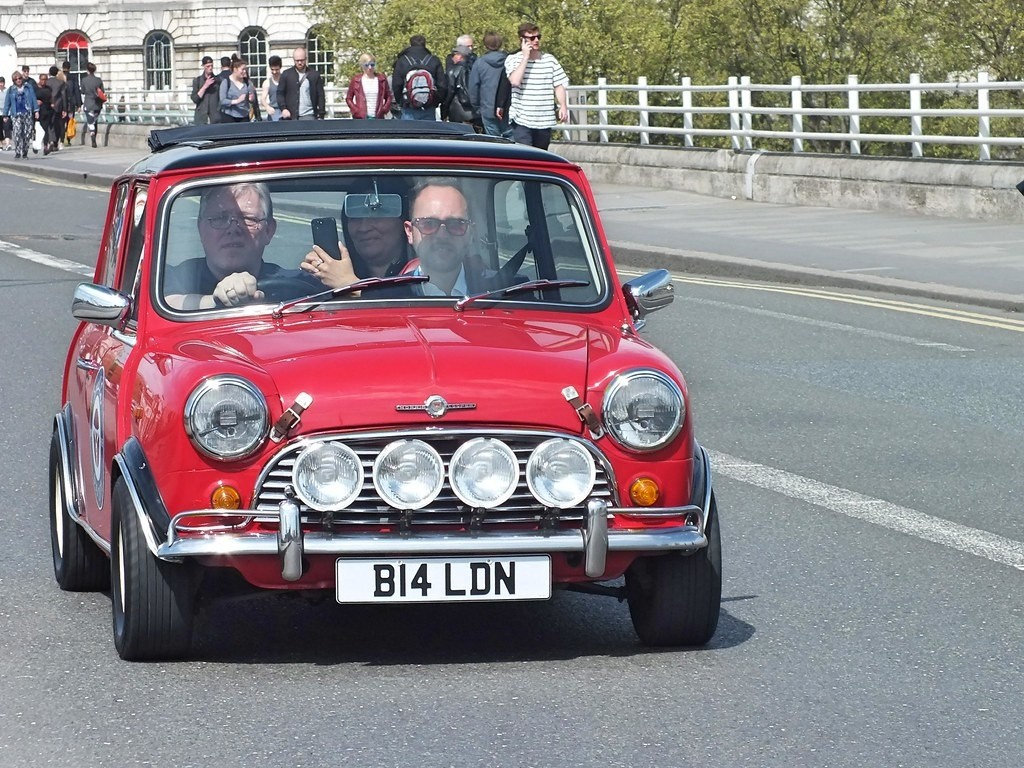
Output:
<box><xmin>96</xmin><ymin>77</ymin><xmax>107</xmax><ymax>102</ymax></box>
<box><xmin>67</xmin><ymin>112</ymin><xmax>76</xmax><ymax>140</ymax></box>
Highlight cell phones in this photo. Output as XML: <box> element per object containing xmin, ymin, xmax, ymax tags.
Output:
<box><xmin>520</xmin><ymin>38</ymin><xmax>533</xmax><ymax>50</ymax></box>
<box><xmin>311</xmin><ymin>216</ymin><xmax>341</xmax><ymax>261</ymax></box>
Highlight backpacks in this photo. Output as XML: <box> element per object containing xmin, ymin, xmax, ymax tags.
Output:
<box><xmin>402</xmin><ymin>53</ymin><xmax>438</xmax><ymax>110</ymax></box>
<box><xmin>451</xmin><ymin>64</ymin><xmax>472</xmax><ymax>106</ymax></box>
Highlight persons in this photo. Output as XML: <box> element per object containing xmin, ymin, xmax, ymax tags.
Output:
<box><xmin>0</xmin><ymin>62</ymin><xmax>104</xmax><ymax>159</ymax></box>
<box><xmin>345</xmin><ymin>22</ymin><xmax>570</xmax><ymax>150</ymax></box>
<box><xmin>191</xmin><ymin>46</ymin><xmax>327</xmax><ymax>125</ymax></box>
<box><xmin>163</xmin><ymin>177</ymin><xmax>539</xmax><ymax>311</ymax></box>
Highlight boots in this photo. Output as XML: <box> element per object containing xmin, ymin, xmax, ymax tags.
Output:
<box><xmin>91</xmin><ymin>135</ymin><xmax>97</xmax><ymax>148</ymax></box>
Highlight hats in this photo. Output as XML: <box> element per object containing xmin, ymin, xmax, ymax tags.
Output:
<box><xmin>22</xmin><ymin>66</ymin><xmax>30</xmax><ymax>71</ymax></box>
<box><xmin>201</xmin><ymin>56</ymin><xmax>213</xmax><ymax>66</ymax></box>
<box><xmin>452</xmin><ymin>44</ymin><xmax>472</xmax><ymax>57</ymax></box>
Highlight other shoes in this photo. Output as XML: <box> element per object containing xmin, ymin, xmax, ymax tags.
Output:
<box><xmin>0</xmin><ymin>139</ymin><xmax>72</xmax><ymax>160</ymax></box>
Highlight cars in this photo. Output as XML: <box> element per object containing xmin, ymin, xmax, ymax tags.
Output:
<box><xmin>47</xmin><ymin>117</ymin><xmax>723</xmax><ymax>663</ymax></box>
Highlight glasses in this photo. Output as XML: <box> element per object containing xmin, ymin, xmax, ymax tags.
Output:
<box><xmin>410</xmin><ymin>218</ymin><xmax>472</xmax><ymax>236</ymax></box>
<box><xmin>362</xmin><ymin>61</ymin><xmax>376</xmax><ymax>68</ymax></box>
<box><xmin>522</xmin><ymin>34</ymin><xmax>542</xmax><ymax>42</ymax></box>
<box><xmin>13</xmin><ymin>76</ymin><xmax>22</xmax><ymax>82</ymax></box>
<box><xmin>199</xmin><ymin>214</ymin><xmax>266</xmax><ymax>229</ymax></box>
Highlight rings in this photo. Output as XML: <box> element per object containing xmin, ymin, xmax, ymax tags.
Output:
<box><xmin>315</xmin><ymin>259</ymin><xmax>323</xmax><ymax>267</ymax></box>
<box><xmin>226</xmin><ymin>288</ymin><xmax>234</xmax><ymax>293</ymax></box>
